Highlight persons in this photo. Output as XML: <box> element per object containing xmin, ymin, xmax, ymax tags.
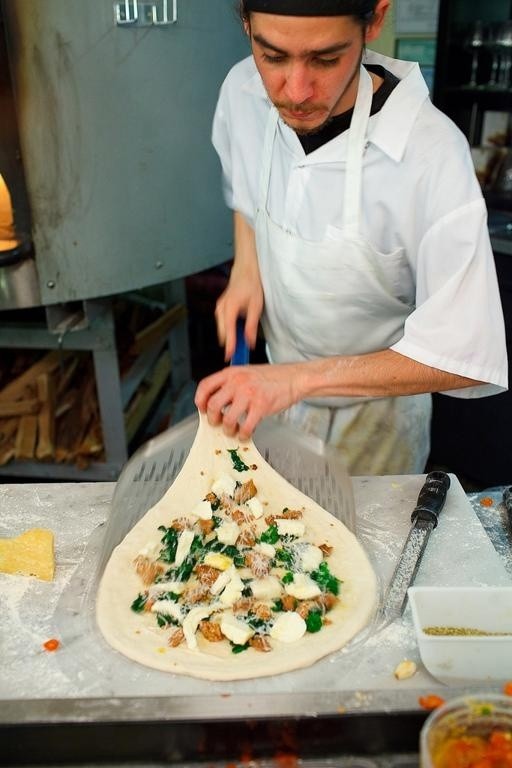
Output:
<box><xmin>188</xmin><ymin>1</ymin><xmax>509</xmax><ymax>474</ymax></box>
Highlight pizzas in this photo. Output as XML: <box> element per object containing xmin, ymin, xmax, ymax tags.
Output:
<box><xmin>95</xmin><ymin>408</ymin><xmax>379</xmax><ymax>682</ymax></box>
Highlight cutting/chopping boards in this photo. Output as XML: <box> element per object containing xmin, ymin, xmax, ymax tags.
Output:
<box><xmin>1</xmin><ymin>471</ymin><xmax>511</xmax><ymax>724</ymax></box>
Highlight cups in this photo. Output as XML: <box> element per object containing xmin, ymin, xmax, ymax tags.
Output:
<box><xmin>419</xmin><ymin>696</ymin><xmax>509</xmax><ymax>768</ymax></box>
